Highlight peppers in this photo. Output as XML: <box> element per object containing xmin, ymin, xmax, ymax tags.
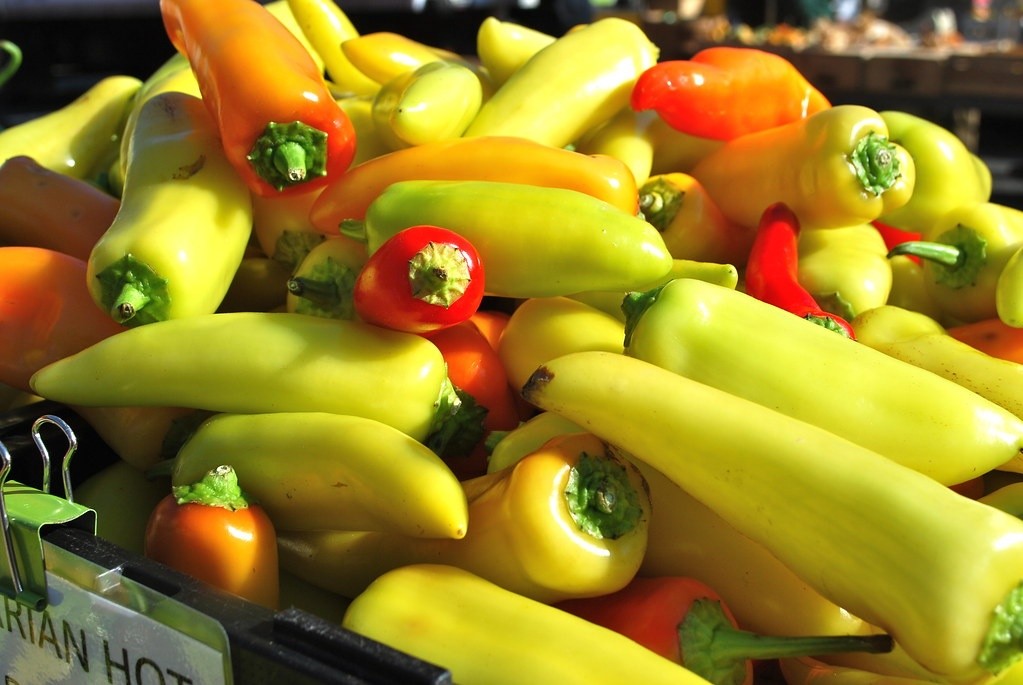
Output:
<box><xmin>0</xmin><ymin>0</ymin><xmax>1023</xmax><ymax>685</ymax></box>
